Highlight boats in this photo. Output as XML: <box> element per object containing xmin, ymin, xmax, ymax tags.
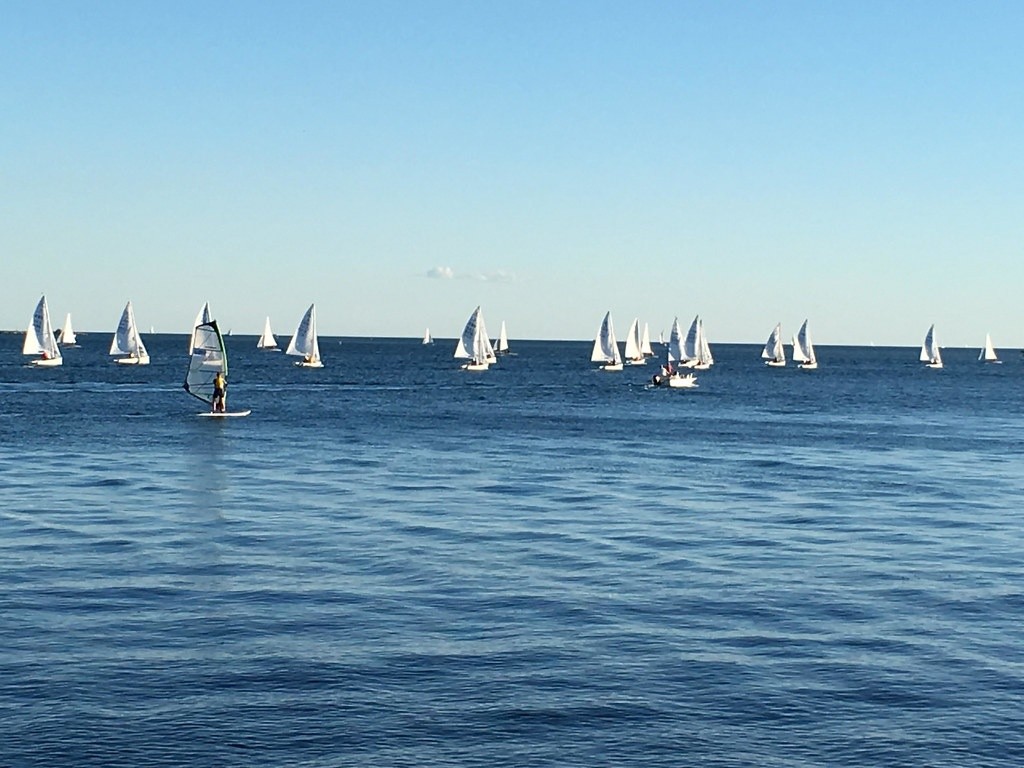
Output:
<box><xmin>654</xmin><ymin>370</ymin><xmax>698</xmax><ymax>388</ymax></box>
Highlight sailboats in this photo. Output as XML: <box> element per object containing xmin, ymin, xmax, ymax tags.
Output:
<box><xmin>479</xmin><ymin>317</ymin><xmax>496</xmax><ymax>363</ymax></box>
<box><xmin>590</xmin><ymin>311</ymin><xmax>623</xmax><ymax>372</ymax></box>
<box><xmin>22</xmin><ymin>295</ymin><xmax>63</xmax><ymax>366</ymax></box>
<box><xmin>107</xmin><ymin>301</ymin><xmax>151</xmax><ymax>365</ymax></box>
<box><xmin>453</xmin><ymin>306</ymin><xmax>490</xmax><ymax>370</ymax></box>
<box><xmin>641</xmin><ymin>322</ymin><xmax>659</xmax><ymax>359</ymax></box>
<box><xmin>185</xmin><ymin>319</ymin><xmax>253</xmax><ymax>418</ymax></box>
<box><xmin>791</xmin><ymin>334</ymin><xmax>810</xmax><ymax>369</ymax></box>
<box><xmin>919</xmin><ymin>324</ymin><xmax>943</xmax><ymax>369</ymax></box>
<box><xmin>800</xmin><ymin>318</ymin><xmax>818</xmax><ymax>370</ymax></box>
<box><xmin>685</xmin><ymin>317</ymin><xmax>714</xmax><ymax>370</ymax></box>
<box><xmin>759</xmin><ymin>322</ymin><xmax>787</xmax><ymax>367</ymax></box>
<box><xmin>659</xmin><ymin>328</ymin><xmax>666</xmax><ymax>344</ymax></box>
<box><xmin>284</xmin><ymin>303</ymin><xmax>326</xmax><ymax>368</ymax></box>
<box><xmin>255</xmin><ymin>315</ymin><xmax>282</xmax><ymax>352</ymax></box>
<box><xmin>56</xmin><ymin>313</ymin><xmax>82</xmax><ymax>348</ymax></box>
<box><xmin>668</xmin><ymin>316</ymin><xmax>699</xmax><ymax>368</ymax></box>
<box><xmin>190</xmin><ymin>302</ymin><xmax>223</xmax><ymax>367</ymax></box>
<box><xmin>623</xmin><ymin>318</ymin><xmax>646</xmax><ymax>365</ymax></box>
<box><xmin>492</xmin><ymin>321</ymin><xmax>510</xmax><ymax>357</ymax></box>
<box><xmin>422</xmin><ymin>328</ymin><xmax>434</xmax><ymax>344</ymax></box>
<box><xmin>978</xmin><ymin>332</ymin><xmax>1003</xmax><ymax>365</ymax></box>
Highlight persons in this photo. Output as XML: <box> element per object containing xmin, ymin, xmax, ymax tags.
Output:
<box><xmin>212</xmin><ymin>371</ymin><xmax>225</xmax><ymax>413</ymax></box>
